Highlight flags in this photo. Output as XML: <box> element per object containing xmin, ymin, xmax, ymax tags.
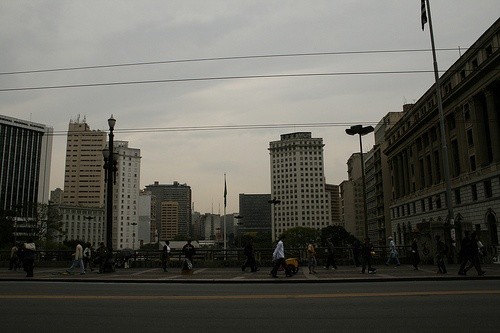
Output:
<box><xmin>224</xmin><ymin>181</ymin><xmax>227</xmax><ymax>207</ymax></box>
<box><xmin>421</xmin><ymin>0</ymin><xmax>427</xmax><ymax>30</ymax></box>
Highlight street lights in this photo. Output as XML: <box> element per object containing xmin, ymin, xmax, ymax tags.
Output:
<box><xmin>101</xmin><ymin>114</ymin><xmax>119</xmax><ymax>259</ymax></box>
<box><xmin>345</xmin><ymin>124</ymin><xmax>376</xmax><ymax>240</ymax></box>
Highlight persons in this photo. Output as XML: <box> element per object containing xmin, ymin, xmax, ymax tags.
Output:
<box><xmin>66</xmin><ymin>240</ymin><xmax>87</xmax><ymax>275</ymax></box>
<box><xmin>271</xmin><ymin>236</ymin><xmax>290</xmax><ymax>278</ymax></box>
<box><xmin>163</xmin><ymin>240</ymin><xmax>172</xmax><ymax>272</ymax></box>
<box><xmin>11</xmin><ymin>241</ymin><xmax>35</xmax><ymax>276</ymax></box>
<box><xmin>435</xmin><ymin>234</ymin><xmax>447</xmax><ymax>274</ymax></box>
<box><xmin>410</xmin><ymin>236</ymin><xmax>420</xmax><ymax>270</ymax></box>
<box><xmin>84</xmin><ymin>243</ymin><xmax>94</xmax><ymax>272</ymax></box>
<box><xmin>323</xmin><ymin>240</ymin><xmax>337</xmax><ymax>270</ymax></box>
<box><xmin>182</xmin><ymin>240</ymin><xmax>196</xmax><ymax>265</ymax></box>
<box><xmin>308</xmin><ymin>240</ymin><xmax>318</xmax><ymax>274</ymax></box>
<box><xmin>385</xmin><ymin>236</ymin><xmax>400</xmax><ymax>265</ymax></box>
<box><xmin>97</xmin><ymin>242</ymin><xmax>107</xmax><ymax>273</ymax></box>
<box><xmin>458</xmin><ymin>232</ymin><xmax>485</xmax><ymax>275</ymax></box>
<box><xmin>242</xmin><ymin>240</ymin><xmax>257</xmax><ymax>272</ymax></box>
<box><xmin>352</xmin><ymin>237</ymin><xmax>375</xmax><ymax>274</ymax></box>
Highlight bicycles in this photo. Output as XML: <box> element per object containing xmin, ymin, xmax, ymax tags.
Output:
<box><xmin>284</xmin><ymin>252</ymin><xmax>299</xmax><ymax>276</ymax></box>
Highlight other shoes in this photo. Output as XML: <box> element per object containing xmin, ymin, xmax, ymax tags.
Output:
<box><xmin>464</xmin><ymin>268</ymin><xmax>468</xmax><ymax>276</ymax></box>
<box><xmin>479</xmin><ymin>270</ymin><xmax>486</xmax><ymax>276</ymax></box>
